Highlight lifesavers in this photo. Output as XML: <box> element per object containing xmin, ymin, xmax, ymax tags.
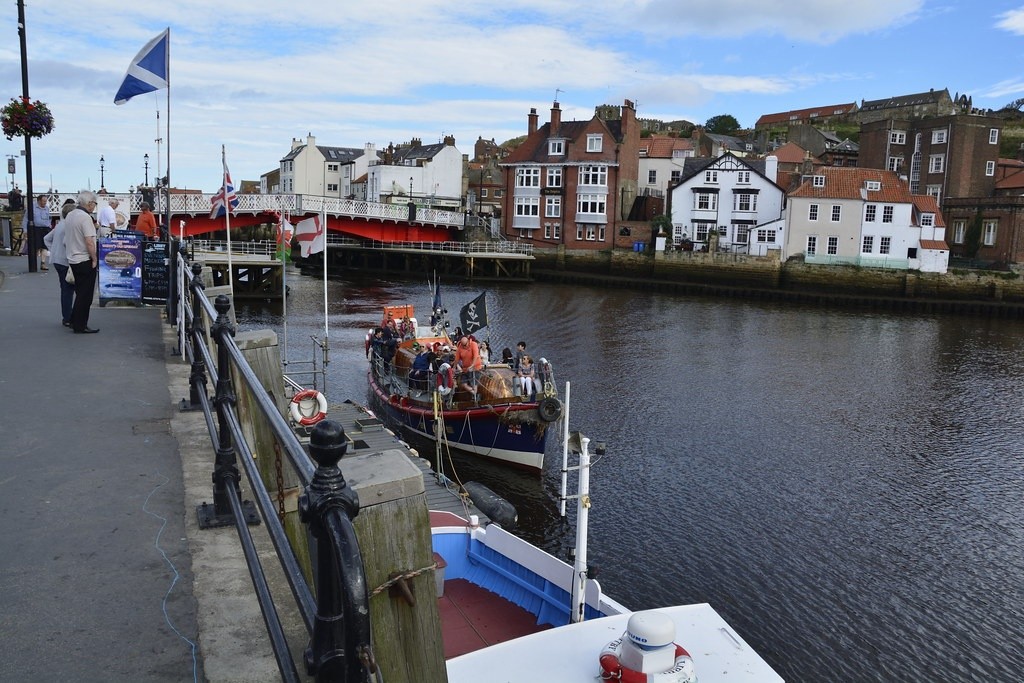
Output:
<box><xmin>539</xmin><ymin>397</ymin><xmax>562</xmax><ymax>422</ymax></box>
<box><xmin>437</xmin><ymin>362</ymin><xmax>454</xmax><ymax>395</ymax></box>
<box><xmin>598</xmin><ymin>636</ymin><xmax>695</xmax><ymax>683</ymax></box>
<box><xmin>365</xmin><ymin>328</ymin><xmax>374</xmax><ymax>356</ymax></box>
<box><xmin>290</xmin><ymin>389</ymin><xmax>328</xmax><ymax>426</ymax></box>
<box><xmin>426</xmin><ymin>341</ymin><xmax>449</xmax><ymax>351</ymax></box>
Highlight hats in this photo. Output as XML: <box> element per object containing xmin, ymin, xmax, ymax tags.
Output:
<box><xmin>61</xmin><ymin>198</ymin><xmax>75</xmax><ymax>207</ymax></box>
<box><xmin>449</xmin><ymin>354</ymin><xmax>456</xmax><ymax>360</ymax></box>
<box><xmin>139</xmin><ymin>202</ymin><xmax>150</xmax><ymax>210</ymax></box>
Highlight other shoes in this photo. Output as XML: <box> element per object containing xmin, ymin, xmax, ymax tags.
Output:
<box><xmin>41</xmin><ymin>263</ymin><xmax>49</xmax><ymax>270</ymax></box>
<box><xmin>69</xmin><ymin>323</ymin><xmax>74</xmax><ymax>330</ymax></box>
<box><xmin>75</xmin><ymin>326</ymin><xmax>100</xmax><ymax>334</ymax></box>
<box><xmin>416</xmin><ymin>390</ymin><xmax>423</xmax><ymax>397</ymax></box>
<box><xmin>471</xmin><ymin>394</ymin><xmax>480</xmax><ymax>403</ymax></box>
<box><xmin>62</xmin><ymin>318</ymin><xmax>69</xmax><ymax>326</ymax></box>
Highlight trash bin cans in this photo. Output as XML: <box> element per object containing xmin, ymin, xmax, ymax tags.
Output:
<box><xmin>639</xmin><ymin>242</ymin><xmax>645</xmax><ymax>252</ymax></box>
<box><xmin>0</xmin><ymin>216</ymin><xmax>14</xmax><ymax>255</ymax></box>
<box><xmin>633</xmin><ymin>242</ymin><xmax>638</xmax><ymax>252</ymax></box>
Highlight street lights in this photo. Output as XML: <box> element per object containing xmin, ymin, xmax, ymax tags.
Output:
<box><xmin>144</xmin><ymin>153</ymin><xmax>149</xmax><ymax>186</ymax></box>
<box><xmin>409</xmin><ymin>176</ymin><xmax>414</xmax><ymax>203</ymax></box>
<box><xmin>100</xmin><ymin>158</ymin><xmax>105</xmax><ymax>189</ymax></box>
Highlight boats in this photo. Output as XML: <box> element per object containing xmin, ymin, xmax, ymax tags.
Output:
<box><xmin>365</xmin><ymin>303</ymin><xmax>565</xmax><ymax>477</ymax></box>
<box><xmin>428</xmin><ymin>436</ymin><xmax>786</xmax><ymax>681</ymax></box>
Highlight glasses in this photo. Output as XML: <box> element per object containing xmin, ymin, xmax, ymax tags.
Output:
<box><xmin>449</xmin><ymin>359</ymin><xmax>455</xmax><ymax>362</ymax></box>
<box><xmin>461</xmin><ymin>342</ymin><xmax>468</xmax><ymax>346</ymax></box>
<box><xmin>419</xmin><ymin>345</ymin><xmax>425</xmax><ymax>348</ymax></box>
<box><xmin>88</xmin><ymin>200</ymin><xmax>98</xmax><ymax>206</ymax></box>
<box><xmin>523</xmin><ymin>358</ymin><xmax>526</xmax><ymax>360</ymax></box>
<box><xmin>396</xmin><ymin>341</ymin><xmax>400</xmax><ymax>344</ymax></box>
<box><xmin>388</xmin><ymin>314</ymin><xmax>393</xmax><ymax>317</ymax></box>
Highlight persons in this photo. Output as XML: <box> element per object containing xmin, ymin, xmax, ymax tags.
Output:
<box><xmin>373</xmin><ymin>314</ymin><xmax>414</xmax><ymax>371</ymax></box>
<box><xmin>23</xmin><ymin>195</ymin><xmax>52</xmax><ymax>271</ymax></box>
<box><xmin>410</xmin><ymin>327</ymin><xmax>491</xmax><ymax>401</ymax></box>
<box><xmin>99</xmin><ymin>199</ymin><xmax>118</xmax><ymax>230</ymax></box>
<box><xmin>135</xmin><ymin>202</ymin><xmax>155</xmax><ymax>240</ymax></box>
<box><xmin>503</xmin><ymin>342</ymin><xmax>535</xmax><ymax>395</ymax></box>
<box><xmin>61</xmin><ymin>199</ymin><xmax>75</xmax><ymax>207</ymax></box>
<box><xmin>63</xmin><ymin>191</ymin><xmax>100</xmax><ymax>333</ymax></box>
<box><xmin>43</xmin><ymin>203</ymin><xmax>73</xmax><ymax>326</ymax></box>
<box><xmin>96</xmin><ymin>189</ymin><xmax>107</xmax><ymax>194</ymax></box>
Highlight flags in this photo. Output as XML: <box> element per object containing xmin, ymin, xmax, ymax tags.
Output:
<box><xmin>275</xmin><ymin>214</ymin><xmax>294</xmax><ymax>262</ymax></box>
<box><xmin>296</xmin><ymin>214</ymin><xmax>324</xmax><ymax>258</ymax></box>
<box><xmin>460</xmin><ymin>293</ymin><xmax>488</xmax><ymax>334</ymax></box>
<box><xmin>209</xmin><ymin>167</ymin><xmax>239</xmax><ymax>219</ymax></box>
<box><xmin>434</xmin><ymin>285</ymin><xmax>441</xmax><ymax>308</ymax></box>
<box><xmin>114</xmin><ymin>28</ymin><xmax>168</xmax><ymax>105</ymax></box>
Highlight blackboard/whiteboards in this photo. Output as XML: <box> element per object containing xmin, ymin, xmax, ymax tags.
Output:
<box><xmin>141</xmin><ymin>241</ymin><xmax>170</xmax><ymax>300</ymax></box>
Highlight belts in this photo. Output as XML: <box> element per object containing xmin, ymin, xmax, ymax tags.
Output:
<box><xmin>100</xmin><ymin>225</ymin><xmax>111</xmax><ymax>228</ymax></box>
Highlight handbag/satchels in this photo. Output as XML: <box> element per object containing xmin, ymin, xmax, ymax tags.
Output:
<box><xmin>65</xmin><ymin>264</ymin><xmax>75</xmax><ymax>284</ymax></box>
